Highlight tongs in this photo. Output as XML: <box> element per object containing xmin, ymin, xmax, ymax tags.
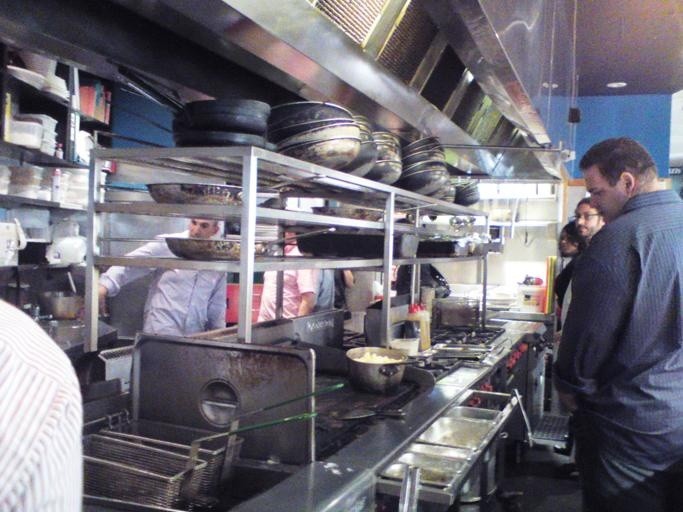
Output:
<box><xmin>504</xmin><ymin>388</ymin><xmax>533</xmax><ymax>448</ymax></box>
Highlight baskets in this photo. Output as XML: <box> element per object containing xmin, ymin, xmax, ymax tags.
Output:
<box><xmin>81</xmin><ymin>417</ymin><xmax>245</xmax><ymax>508</ymax></box>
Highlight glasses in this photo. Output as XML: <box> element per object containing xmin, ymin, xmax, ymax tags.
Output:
<box><xmin>572</xmin><ymin>213</ymin><xmax>600</xmax><ymax>221</ymax></box>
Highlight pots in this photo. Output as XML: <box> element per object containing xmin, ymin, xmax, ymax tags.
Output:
<box><xmin>346</xmin><ymin>346</ymin><xmax>425</xmax><ymax>396</ymax></box>
<box><xmin>165</xmin><ymin>227</ymin><xmax>337</xmax><ymax>261</ymax></box>
<box><xmin>311</xmin><ymin>203</ymin><xmax>438</xmax><ymax>223</ymax></box>
<box><xmin>93</xmin><ymin>63</ymin><xmax>479</xmax><ymax>207</ymax></box>
<box><xmin>1</xmin><ymin>287</ymin><xmax>84</xmax><ymax>320</ymax></box>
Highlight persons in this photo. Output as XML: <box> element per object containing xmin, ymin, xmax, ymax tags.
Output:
<box><xmin>0</xmin><ymin>299</ymin><xmax>83</xmax><ymax>512</ymax></box>
<box><xmin>99</xmin><ymin>218</ymin><xmax>226</xmax><ymax>334</ymax></box>
<box><xmin>258</xmin><ymin>232</ymin><xmax>353</xmax><ymax>322</ymax></box>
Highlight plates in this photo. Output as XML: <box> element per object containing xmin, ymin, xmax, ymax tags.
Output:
<box><xmin>6</xmin><ymin>64</ymin><xmax>71</xmax><ymax>103</ymax></box>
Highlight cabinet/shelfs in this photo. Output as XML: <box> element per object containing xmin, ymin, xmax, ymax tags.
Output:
<box><xmin>0</xmin><ymin>44</ymin><xmax>111</xmax><ymax>322</ymax></box>
<box><xmin>79</xmin><ymin>144</ymin><xmax>496</xmax><ymax>365</ymax></box>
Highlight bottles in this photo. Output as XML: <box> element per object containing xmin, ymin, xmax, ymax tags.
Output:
<box><xmin>52</xmin><ymin>167</ymin><xmax>62</xmax><ymax>204</ymax></box>
<box><xmin>405</xmin><ymin>303</ymin><xmax>432</xmax><ymax>352</ymax></box>
<box><xmin>55</xmin><ymin>143</ymin><xmax>65</xmax><ymax>161</ymax></box>
